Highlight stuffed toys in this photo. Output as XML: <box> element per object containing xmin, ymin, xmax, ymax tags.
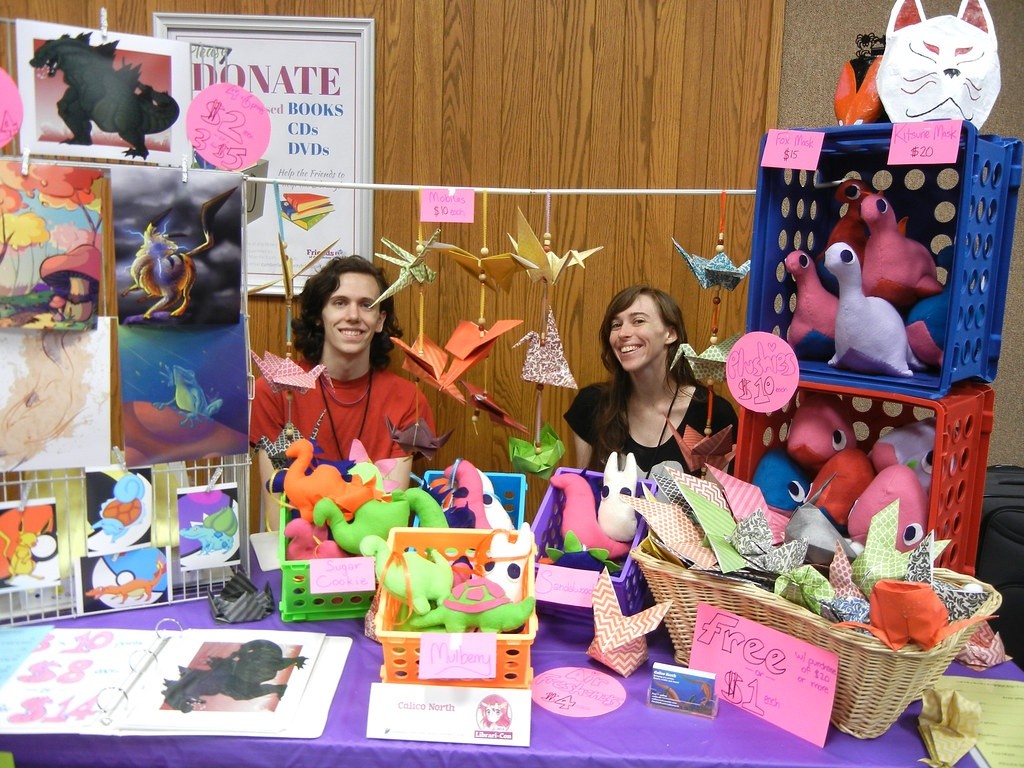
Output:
<box><xmin>748</xmin><ymin>169</ymin><xmax>965</xmax><ymax>571</ymax></box>
<box><xmin>265</xmin><ymin>438</ymin><xmax>641</xmax><ymax>637</ymax></box>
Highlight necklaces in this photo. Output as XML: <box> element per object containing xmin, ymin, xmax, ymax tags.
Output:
<box><xmin>625</xmin><ymin>380</ymin><xmax>679</xmax><ymax>479</ymax></box>
<box><xmin>316</xmin><ymin>367</ymin><xmax>372</xmax><ymax>460</ymax></box>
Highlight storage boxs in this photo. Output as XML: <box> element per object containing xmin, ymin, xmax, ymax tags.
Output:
<box><xmin>413</xmin><ymin>469</ymin><xmax>529</xmax><ymax>532</ymax></box>
<box><xmin>737</xmin><ymin>380</ymin><xmax>995</xmax><ymax>574</ymax></box>
<box><xmin>374</xmin><ymin>528</ymin><xmax>539</xmax><ymax>690</ymax></box>
<box><xmin>744</xmin><ymin>120</ymin><xmax>1023</xmax><ymax>399</ymax></box>
<box><xmin>526</xmin><ymin>465</ymin><xmax>658</xmax><ymax>624</ymax></box>
<box><xmin>276</xmin><ymin>493</ymin><xmax>389</xmax><ymax>625</ymax></box>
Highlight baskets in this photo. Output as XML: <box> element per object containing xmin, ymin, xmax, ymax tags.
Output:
<box><xmin>734</xmin><ymin>121</ymin><xmax>1024</xmax><ymax>584</ymax></box>
<box><xmin>630</xmin><ymin>503</ymin><xmax>1003</xmax><ymax>742</ymax></box>
<box><xmin>530</xmin><ymin>465</ymin><xmax>657</xmax><ymax>629</ymax></box>
<box><xmin>277</xmin><ymin>461</ymin><xmax>538</xmax><ymax>690</ymax></box>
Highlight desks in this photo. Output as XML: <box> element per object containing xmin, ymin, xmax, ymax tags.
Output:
<box><xmin>0</xmin><ymin>531</ymin><xmax>1024</xmax><ymax>767</ymax></box>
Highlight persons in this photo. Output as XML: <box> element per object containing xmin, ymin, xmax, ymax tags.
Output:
<box><xmin>250</xmin><ymin>255</ymin><xmax>436</xmax><ymax>531</ymax></box>
<box><xmin>563</xmin><ymin>284</ymin><xmax>738</xmax><ymax>480</ymax></box>
